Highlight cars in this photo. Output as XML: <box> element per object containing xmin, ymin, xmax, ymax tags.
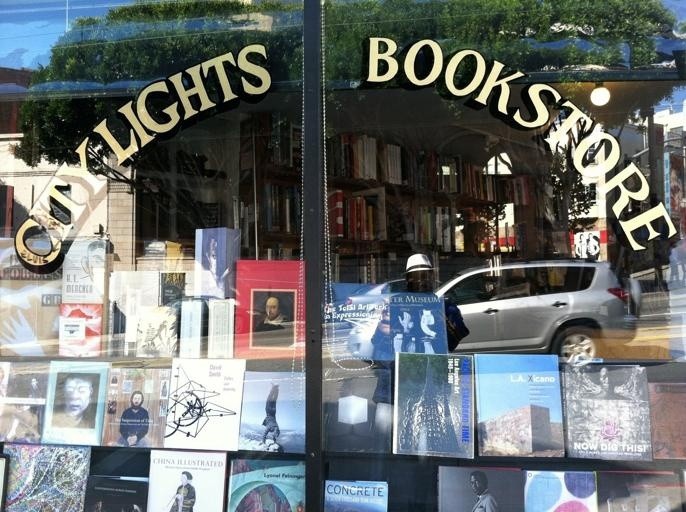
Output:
<box><xmin>344</xmin><ymin>277</ymin><xmax>440</xmax><ymax>321</ymax></box>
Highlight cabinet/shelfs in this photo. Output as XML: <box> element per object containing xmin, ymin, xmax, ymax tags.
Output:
<box><xmin>241</xmin><ymin>115</ymin><xmax>573</xmax><ymax>297</ymax></box>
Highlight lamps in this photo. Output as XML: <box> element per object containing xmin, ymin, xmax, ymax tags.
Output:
<box><xmin>589</xmin><ymin>81</ymin><xmax>611</xmax><ymax>107</ymax></box>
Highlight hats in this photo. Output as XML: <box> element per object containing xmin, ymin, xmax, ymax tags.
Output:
<box><xmin>403</xmin><ymin>254</ymin><xmax>437</xmax><ymax>273</ymax></box>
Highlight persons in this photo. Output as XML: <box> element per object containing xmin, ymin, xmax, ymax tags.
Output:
<box><xmin>1</xmin><ymin>110</ymin><xmax>686</xmax><ymax>509</ymax></box>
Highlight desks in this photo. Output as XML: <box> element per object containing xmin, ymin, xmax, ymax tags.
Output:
<box><xmin>135</xmin><ymin>251</ymin><xmax>204</xmax><ymax>305</ymax></box>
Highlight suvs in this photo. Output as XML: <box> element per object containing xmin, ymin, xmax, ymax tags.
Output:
<box><xmin>345</xmin><ymin>254</ymin><xmax>642</xmax><ymax>372</ymax></box>
